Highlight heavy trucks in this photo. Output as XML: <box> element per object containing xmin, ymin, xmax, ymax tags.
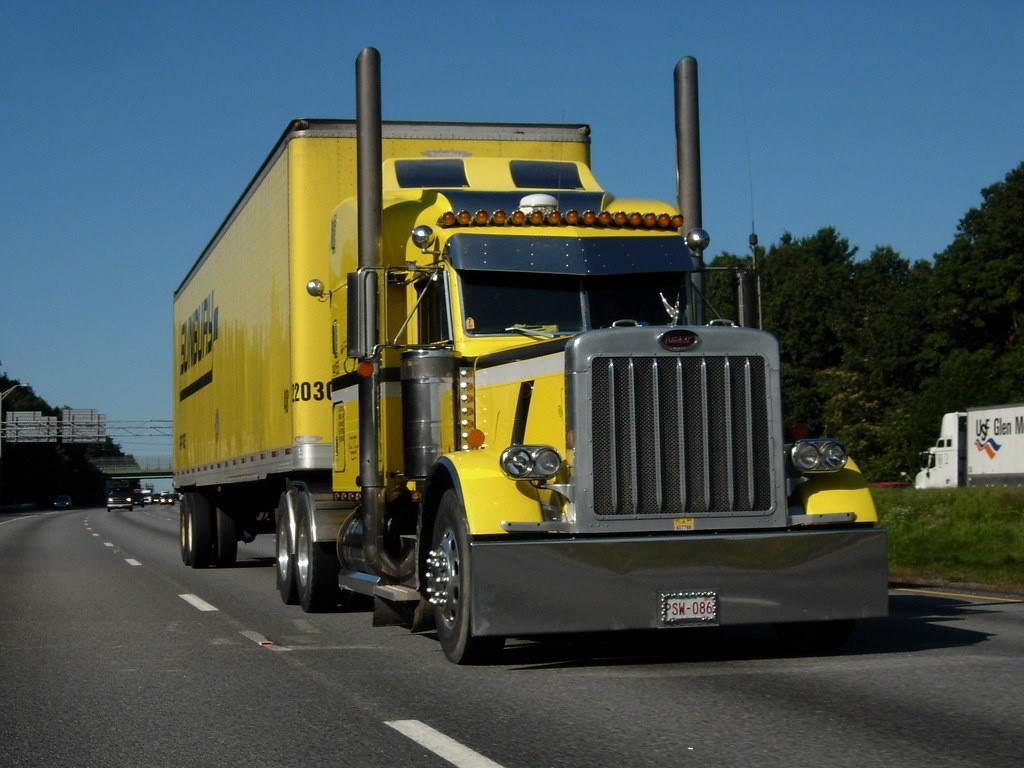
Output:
<box><xmin>172</xmin><ymin>46</ymin><xmax>889</xmax><ymax>666</ymax></box>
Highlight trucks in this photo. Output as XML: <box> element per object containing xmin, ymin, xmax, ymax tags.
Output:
<box><xmin>914</xmin><ymin>402</ymin><xmax>1024</xmax><ymax>490</ymax></box>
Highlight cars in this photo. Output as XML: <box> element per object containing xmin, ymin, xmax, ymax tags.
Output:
<box><xmin>132</xmin><ymin>487</ymin><xmax>175</xmax><ymax>507</ymax></box>
<box><xmin>53</xmin><ymin>495</ymin><xmax>73</xmax><ymax>510</ymax></box>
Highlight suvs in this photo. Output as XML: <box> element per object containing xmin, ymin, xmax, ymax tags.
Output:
<box><xmin>105</xmin><ymin>485</ymin><xmax>134</xmax><ymax>512</ymax></box>
